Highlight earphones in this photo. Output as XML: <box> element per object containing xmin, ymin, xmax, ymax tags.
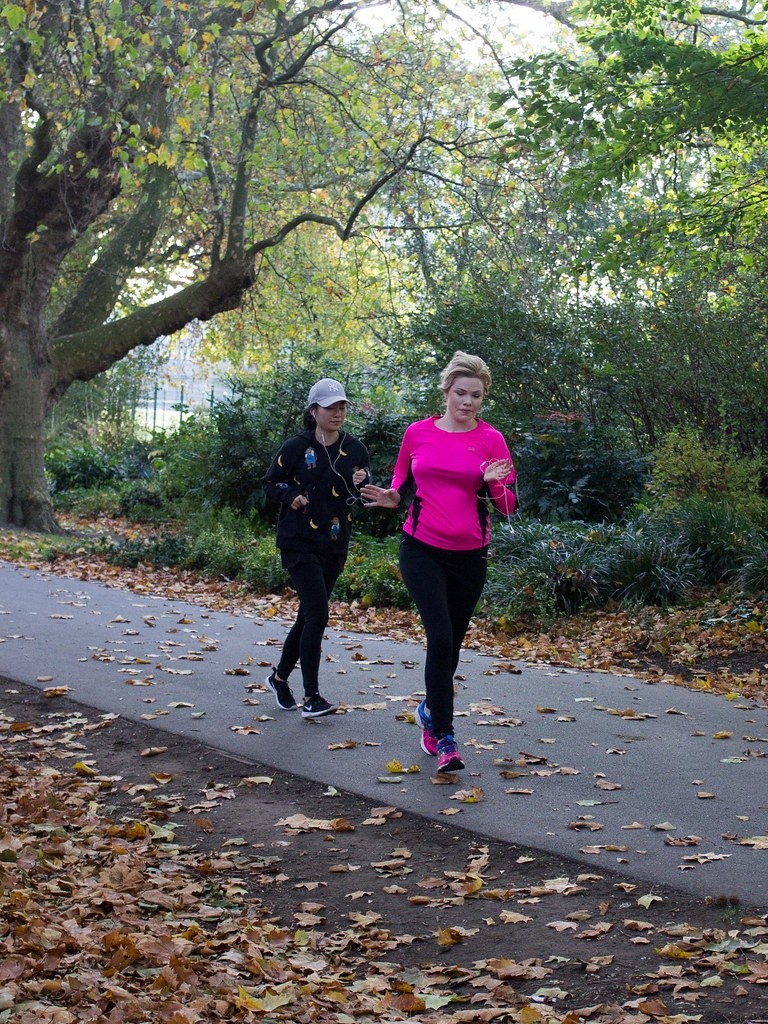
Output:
<box><xmin>509</xmin><ymin>525</ymin><xmax>514</xmax><ymax>534</ymax></box>
<box><xmin>312</xmin><ymin>410</ymin><xmax>316</xmax><ymax>417</ymax></box>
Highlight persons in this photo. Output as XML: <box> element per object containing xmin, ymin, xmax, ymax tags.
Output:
<box><xmin>360</xmin><ymin>351</ymin><xmax>518</xmax><ymax>772</ymax></box>
<box><xmin>264</xmin><ymin>377</ymin><xmax>370</xmax><ymax>718</ymax></box>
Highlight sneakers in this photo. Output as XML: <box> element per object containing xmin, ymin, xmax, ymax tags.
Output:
<box><xmin>436</xmin><ymin>735</ymin><xmax>466</xmax><ymax>774</ymax></box>
<box><xmin>302</xmin><ymin>693</ymin><xmax>337</xmax><ymax>719</ymax></box>
<box><xmin>414</xmin><ymin>699</ymin><xmax>437</xmax><ymax>756</ymax></box>
<box><xmin>266</xmin><ymin>666</ymin><xmax>298</xmax><ymax>710</ymax></box>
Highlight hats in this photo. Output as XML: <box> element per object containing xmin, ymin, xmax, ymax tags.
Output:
<box><xmin>308</xmin><ymin>378</ymin><xmax>353</xmax><ymax>408</ymax></box>
